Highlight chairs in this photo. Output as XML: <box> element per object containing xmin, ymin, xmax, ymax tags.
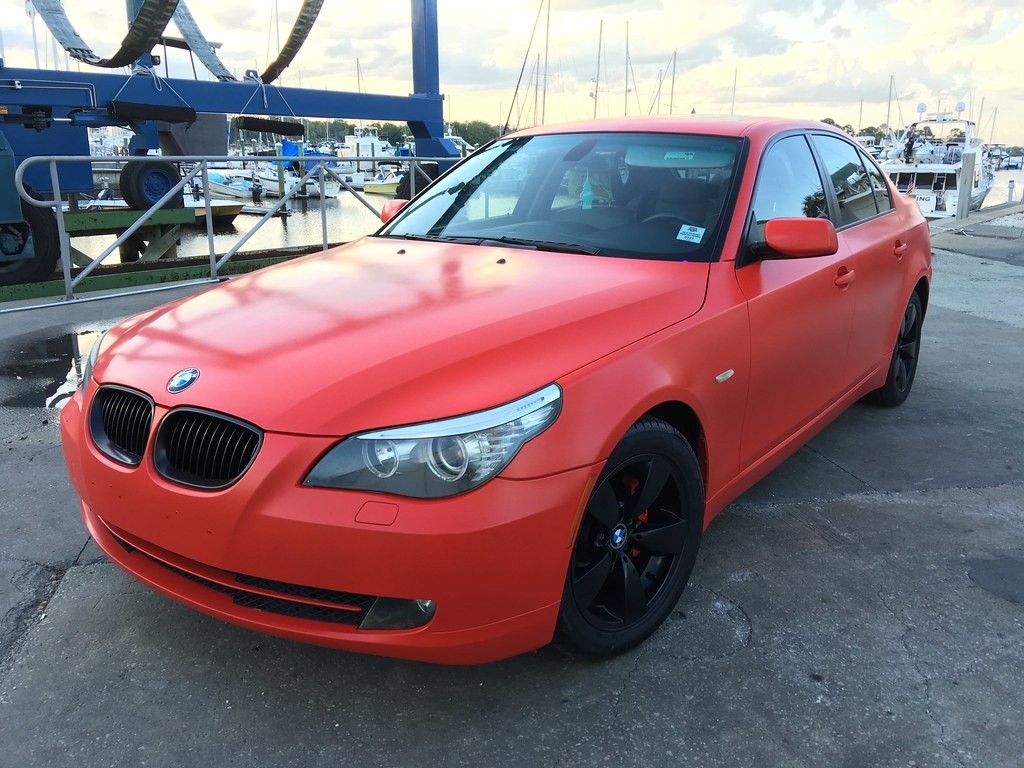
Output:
<box><xmin>570</xmin><ymin>168</ymin><xmax>636</xmax><ymax>232</ymax></box>
<box><xmin>656</xmin><ymin>177</ymin><xmax>711</xmax><ymax>222</ymax></box>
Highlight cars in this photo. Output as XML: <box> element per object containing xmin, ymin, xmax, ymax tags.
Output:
<box><xmin>60</xmin><ymin>118</ymin><xmax>932</xmax><ymax>662</ymax></box>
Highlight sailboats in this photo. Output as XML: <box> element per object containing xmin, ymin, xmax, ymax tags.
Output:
<box><xmin>852</xmin><ymin>75</ymin><xmax>1011</xmax><ymax>220</ymax></box>
<box><xmin>25</xmin><ymin>0</ymin><xmax>481</xmax><ymax>215</ymax></box>
<box><xmin>488</xmin><ymin>0</ymin><xmax>738</xmax><ymax>196</ymax></box>
